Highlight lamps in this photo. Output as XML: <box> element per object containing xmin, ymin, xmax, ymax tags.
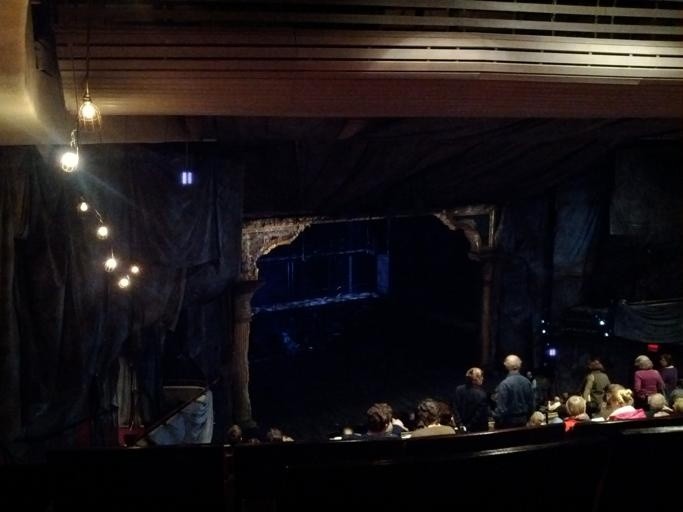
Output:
<box><xmin>60</xmin><ymin>19</ymin><xmax>139</xmax><ymax>289</ymax></box>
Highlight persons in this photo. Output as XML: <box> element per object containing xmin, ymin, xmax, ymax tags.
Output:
<box><xmin>224</xmin><ymin>353</ymin><xmax>683</xmax><ymax>456</ymax></box>
